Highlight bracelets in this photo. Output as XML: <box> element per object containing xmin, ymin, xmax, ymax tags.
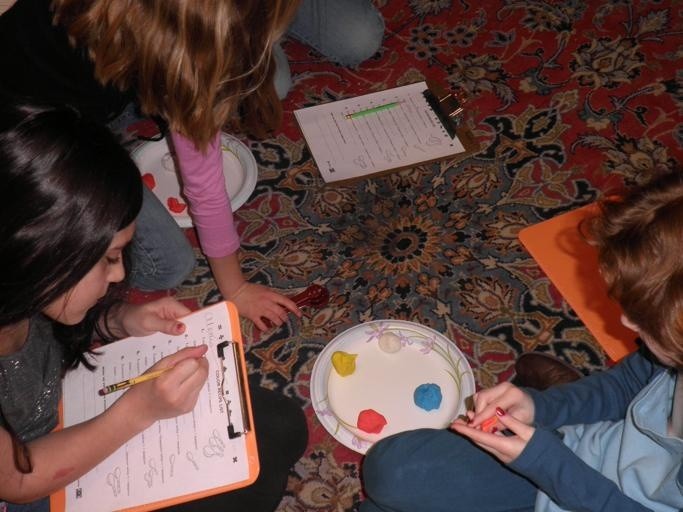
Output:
<box><xmin>90</xmin><ymin>298</ymin><xmax>124</xmax><ymax>343</ymax></box>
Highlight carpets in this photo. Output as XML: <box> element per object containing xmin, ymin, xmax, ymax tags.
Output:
<box><xmin>89</xmin><ymin>0</ymin><xmax>683</xmax><ymax>512</ymax></box>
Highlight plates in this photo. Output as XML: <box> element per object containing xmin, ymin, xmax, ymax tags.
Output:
<box><xmin>311</xmin><ymin>320</ymin><xmax>477</xmax><ymax>457</ymax></box>
<box><xmin>130</xmin><ymin>133</ymin><xmax>258</xmax><ymax>229</ymax></box>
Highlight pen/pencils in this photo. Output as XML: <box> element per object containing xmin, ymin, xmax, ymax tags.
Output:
<box><xmin>97</xmin><ymin>369</ymin><xmax>164</xmax><ymax>396</ymax></box>
<box><xmin>345</xmin><ymin>98</ymin><xmax>406</xmax><ymax>119</ymax></box>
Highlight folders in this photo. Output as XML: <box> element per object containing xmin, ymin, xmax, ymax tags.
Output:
<box><xmin>518</xmin><ymin>196</ymin><xmax>641</xmax><ymax>362</ymax></box>
<box><xmin>48</xmin><ymin>300</ymin><xmax>260</xmax><ymax>510</ymax></box>
<box><xmin>290</xmin><ymin>79</ymin><xmax>481</xmax><ymax>187</ymax></box>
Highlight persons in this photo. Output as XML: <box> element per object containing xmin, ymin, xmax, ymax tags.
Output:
<box><xmin>361</xmin><ymin>171</ymin><xmax>682</xmax><ymax>511</ymax></box>
<box><xmin>267</xmin><ymin>0</ymin><xmax>383</xmax><ymax>101</ymax></box>
<box><xmin>0</xmin><ymin>99</ymin><xmax>308</xmax><ymax>512</ymax></box>
<box><xmin>0</xmin><ymin>0</ymin><xmax>302</xmax><ymax>332</ymax></box>
